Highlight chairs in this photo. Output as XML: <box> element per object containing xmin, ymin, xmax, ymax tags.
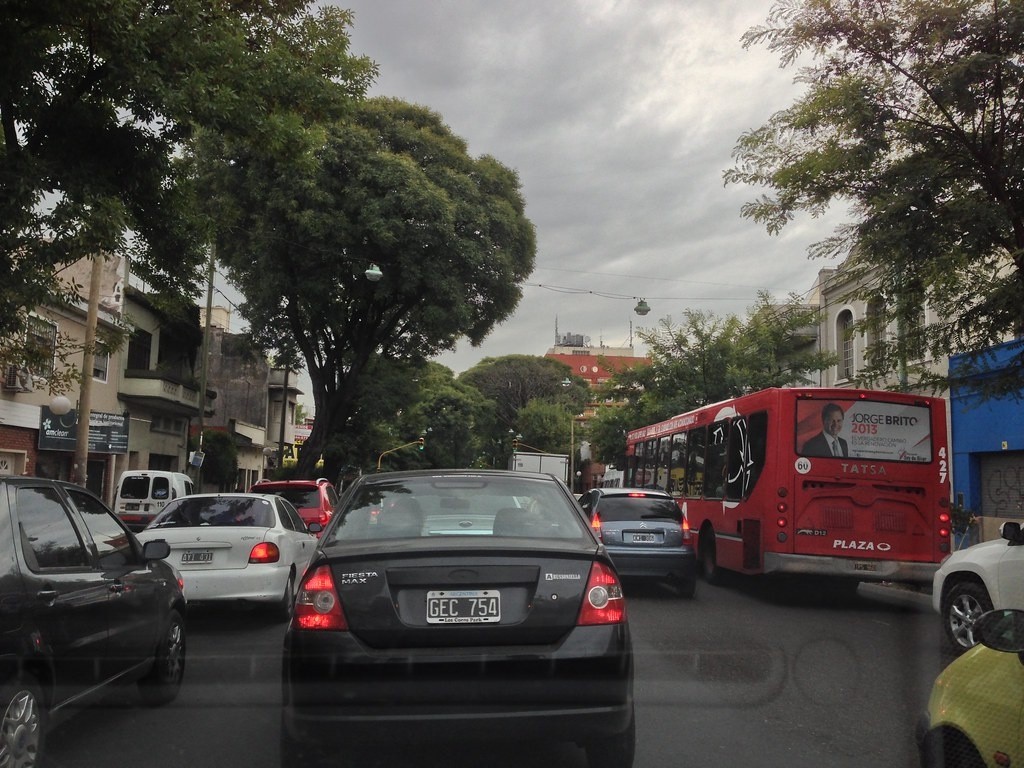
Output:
<box><xmin>376</xmin><ymin>496</ymin><xmax>425</xmax><ymax>538</ymax></box>
<box><xmin>493</xmin><ymin>508</ymin><xmax>538</xmax><ymax>535</ymax></box>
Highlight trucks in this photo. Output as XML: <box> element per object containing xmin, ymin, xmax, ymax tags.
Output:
<box><xmin>508</xmin><ymin>454</ymin><xmax>570</xmax><ymax>489</ymax></box>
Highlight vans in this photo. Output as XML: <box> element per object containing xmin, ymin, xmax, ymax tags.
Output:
<box><xmin>112</xmin><ymin>469</ymin><xmax>196</xmax><ymax>532</ymax></box>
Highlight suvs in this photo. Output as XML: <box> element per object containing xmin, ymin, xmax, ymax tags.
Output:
<box><xmin>248</xmin><ymin>479</ymin><xmax>339</xmax><ymax>538</ymax></box>
<box><xmin>0</xmin><ymin>473</ymin><xmax>188</xmax><ymax>768</ymax></box>
<box><xmin>574</xmin><ymin>487</ymin><xmax>697</xmax><ymax>596</ymax></box>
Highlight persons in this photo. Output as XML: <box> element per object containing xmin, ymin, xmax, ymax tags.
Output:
<box><xmin>802</xmin><ymin>403</ymin><xmax>849</xmax><ymax>458</ymax></box>
<box><xmin>233</xmin><ymin>484</ymin><xmax>246</xmax><ymax>494</ymax></box>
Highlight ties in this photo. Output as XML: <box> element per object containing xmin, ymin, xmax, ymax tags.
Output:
<box><xmin>833</xmin><ymin>440</ymin><xmax>839</xmax><ymax>457</ymax></box>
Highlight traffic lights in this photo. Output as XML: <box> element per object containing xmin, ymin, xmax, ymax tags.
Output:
<box><xmin>419</xmin><ymin>437</ymin><xmax>424</xmax><ymax>449</ymax></box>
<box><xmin>513</xmin><ymin>439</ymin><xmax>518</xmax><ymax>452</ymax></box>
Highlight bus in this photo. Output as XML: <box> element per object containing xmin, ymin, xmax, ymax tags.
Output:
<box><xmin>624</xmin><ymin>385</ymin><xmax>953</xmax><ymax>594</ymax></box>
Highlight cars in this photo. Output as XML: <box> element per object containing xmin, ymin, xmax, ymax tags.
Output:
<box><xmin>916</xmin><ymin>607</ymin><xmax>1024</xmax><ymax>768</ymax></box>
<box><xmin>136</xmin><ymin>494</ymin><xmax>322</xmax><ymax>623</ymax></box>
<box><xmin>278</xmin><ymin>465</ymin><xmax>635</xmax><ymax>767</ymax></box>
<box><xmin>930</xmin><ymin>521</ymin><xmax>1024</xmax><ymax>656</ymax></box>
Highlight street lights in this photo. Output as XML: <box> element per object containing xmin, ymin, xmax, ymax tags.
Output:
<box><xmin>50</xmin><ymin>397</ymin><xmax>89</xmax><ymax>487</ymax></box>
<box><xmin>262</xmin><ymin>448</ymin><xmax>278</xmax><ymax>480</ymax></box>
